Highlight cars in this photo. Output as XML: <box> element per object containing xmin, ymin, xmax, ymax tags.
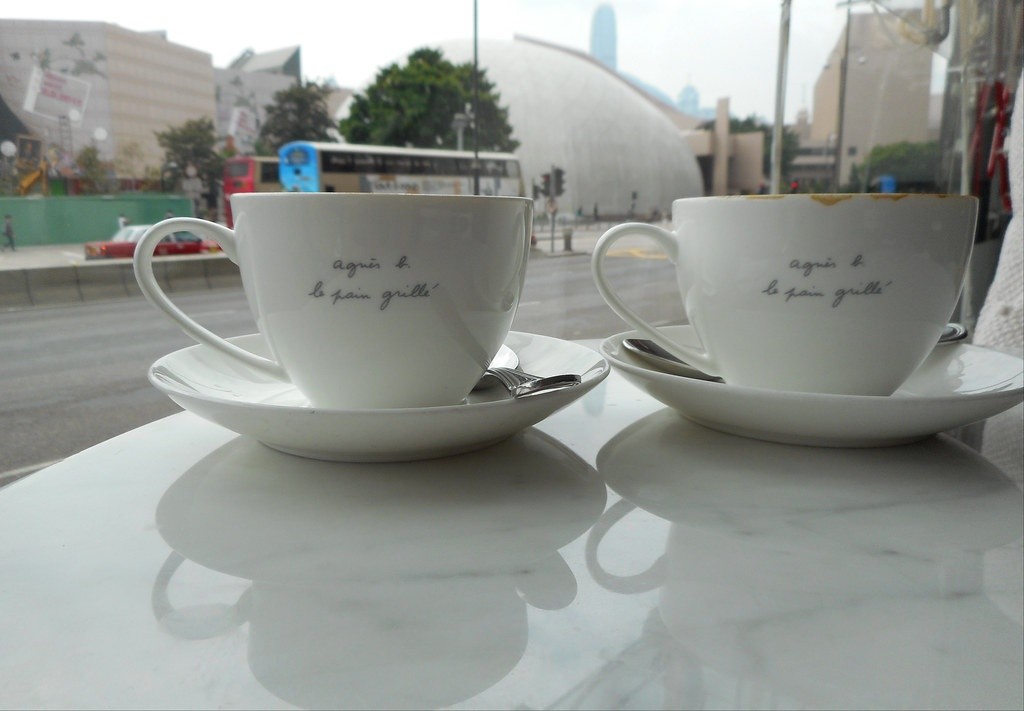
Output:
<box><xmin>85</xmin><ymin>225</ymin><xmax>223</xmax><ymax>259</ymax></box>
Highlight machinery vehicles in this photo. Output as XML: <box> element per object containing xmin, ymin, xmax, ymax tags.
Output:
<box><xmin>16</xmin><ymin>158</ymin><xmax>49</xmax><ymax>196</ymax></box>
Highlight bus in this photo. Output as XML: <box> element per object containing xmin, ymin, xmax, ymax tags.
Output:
<box><xmin>221</xmin><ymin>156</ymin><xmax>283</xmax><ymax>229</ymax></box>
<box><xmin>278</xmin><ymin>140</ymin><xmax>525</xmax><ymax>198</ymax></box>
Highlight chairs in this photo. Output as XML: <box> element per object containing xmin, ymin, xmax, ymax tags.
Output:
<box><xmin>972</xmin><ymin>68</ymin><xmax>1023</xmax><ymax>360</ymax></box>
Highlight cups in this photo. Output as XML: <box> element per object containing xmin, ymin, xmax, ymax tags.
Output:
<box><xmin>591</xmin><ymin>193</ymin><xmax>981</xmax><ymax>396</ymax></box>
<box><xmin>132</xmin><ymin>193</ymin><xmax>533</xmax><ymax>408</ymax></box>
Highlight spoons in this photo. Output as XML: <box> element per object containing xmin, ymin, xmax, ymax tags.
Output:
<box><xmin>622</xmin><ymin>321</ymin><xmax>968</xmax><ymax>383</ymax></box>
<box><xmin>470</xmin><ymin>342</ymin><xmax>580</xmax><ymax>399</ymax></box>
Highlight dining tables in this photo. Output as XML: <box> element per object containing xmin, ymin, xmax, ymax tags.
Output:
<box><xmin>0</xmin><ymin>336</ymin><xmax>1024</xmax><ymax>711</ymax></box>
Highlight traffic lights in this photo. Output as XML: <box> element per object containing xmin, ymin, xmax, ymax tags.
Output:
<box><xmin>555</xmin><ymin>169</ymin><xmax>566</xmax><ymax>194</ymax></box>
<box><xmin>540</xmin><ymin>174</ymin><xmax>549</xmax><ymax>195</ymax></box>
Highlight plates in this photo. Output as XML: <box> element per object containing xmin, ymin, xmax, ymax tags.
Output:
<box><xmin>600</xmin><ymin>324</ymin><xmax>1024</xmax><ymax>446</ymax></box>
<box><xmin>147</xmin><ymin>332</ymin><xmax>610</xmax><ymax>464</ymax></box>
